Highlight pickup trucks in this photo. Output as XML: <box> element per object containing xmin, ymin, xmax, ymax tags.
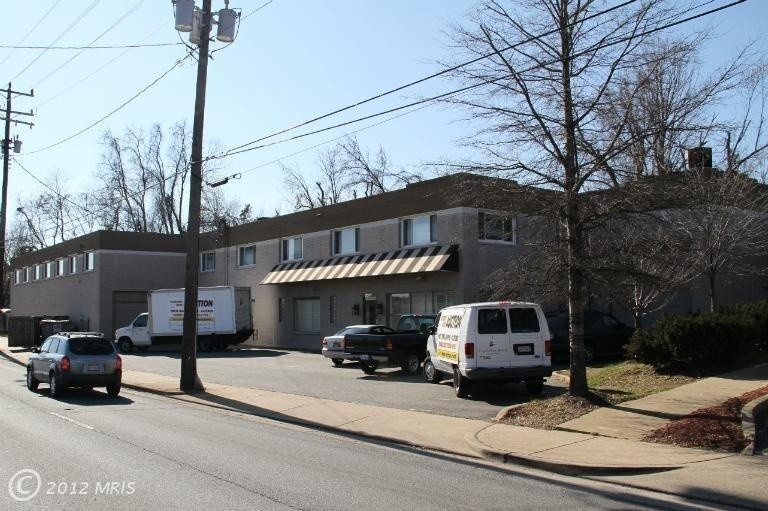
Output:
<box><xmin>342</xmin><ymin>314</ymin><xmax>438</xmax><ymax>376</ymax></box>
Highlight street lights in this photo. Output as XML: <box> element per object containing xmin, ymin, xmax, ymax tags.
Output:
<box><xmin>172</xmin><ymin>1</ymin><xmax>239</xmax><ymax>395</ymax></box>
<box><xmin>0</xmin><ymin>134</ymin><xmax>22</xmax><ymax>280</ymax></box>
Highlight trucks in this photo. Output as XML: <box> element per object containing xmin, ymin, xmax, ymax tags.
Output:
<box><xmin>114</xmin><ymin>286</ymin><xmax>255</xmax><ymax>353</ymax></box>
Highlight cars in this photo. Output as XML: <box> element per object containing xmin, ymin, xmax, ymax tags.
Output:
<box><xmin>26</xmin><ymin>331</ymin><xmax>122</xmax><ymax>400</ymax></box>
<box><xmin>320</xmin><ymin>324</ymin><xmax>397</xmax><ymax>368</ymax></box>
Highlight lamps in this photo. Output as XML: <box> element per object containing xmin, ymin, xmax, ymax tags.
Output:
<box><xmin>351</xmin><ymin>303</ymin><xmax>359</xmax><ymax>314</ymax></box>
<box><xmin>374</xmin><ymin>302</ymin><xmax>383</xmax><ymax>314</ymax></box>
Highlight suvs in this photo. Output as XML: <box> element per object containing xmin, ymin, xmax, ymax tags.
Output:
<box><xmin>548</xmin><ymin>310</ymin><xmax>632</xmax><ymax>366</ymax></box>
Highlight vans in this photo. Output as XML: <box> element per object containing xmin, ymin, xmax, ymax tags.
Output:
<box><xmin>423</xmin><ymin>302</ymin><xmax>553</xmax><ymax>399</ymax></box>
